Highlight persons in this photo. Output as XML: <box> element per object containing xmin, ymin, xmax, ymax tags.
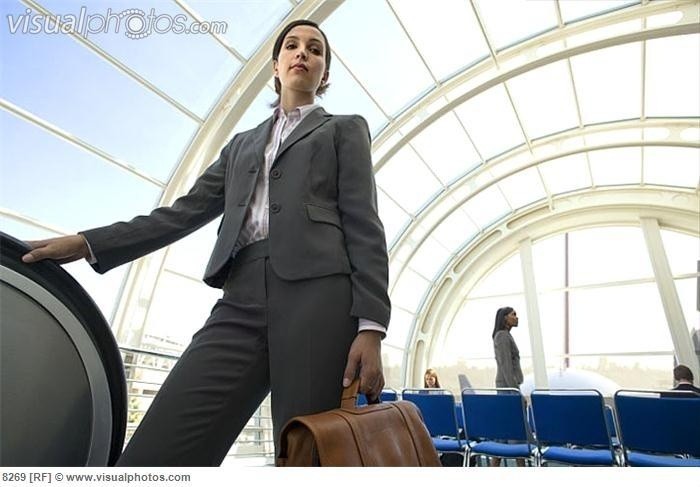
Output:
<box><xmin>661</xmin><ymin>366</ymin><xmax>700</xmax><ymax>398</ymax></box>
<box><xmin>489</xmin><ymin>307</ymin><xmax>525</xmax><ymax>467</ymax></box>
<box><xmin>23</xmin><ymin>19</ymin><xmax>391</xmax><ymax>467</ymax></box>
<box><xmin>418</xmin><ymin>369</ymin><xmax>444</xmax><ymax>395</ymax></box>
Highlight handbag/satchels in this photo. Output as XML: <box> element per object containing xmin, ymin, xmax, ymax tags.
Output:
<box><xmin>274</xmin><ymin>376</ymin><xmax>442</xmax><ymax>467</ymax></box>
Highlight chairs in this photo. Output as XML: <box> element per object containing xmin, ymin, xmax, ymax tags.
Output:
<box><xmin>359</xmin><ymin>384</ymin><xmax>698</xmax><ymax>466</ymax></box>
